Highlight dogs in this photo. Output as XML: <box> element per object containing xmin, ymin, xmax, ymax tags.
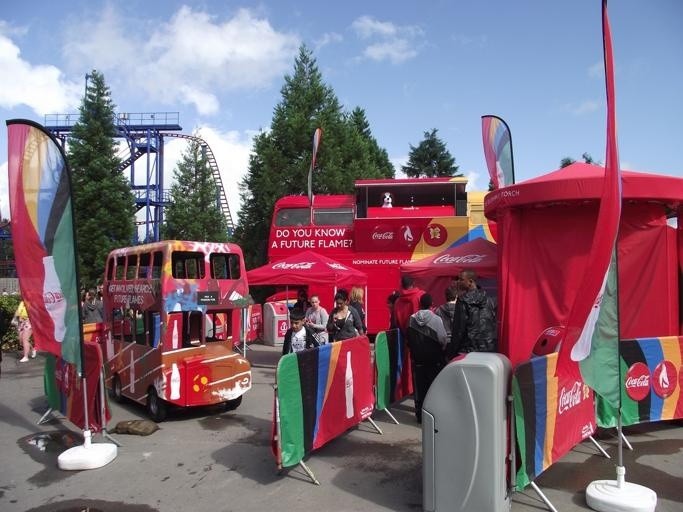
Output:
<box><xmin>380</xmin><ymin>191</ymin><xmax>394</xmax><ymax>208</ymax></box>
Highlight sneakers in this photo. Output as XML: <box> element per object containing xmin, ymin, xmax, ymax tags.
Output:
<box><xmin>19</xmin><ymin>357</ymin><xmax>29</xmax><ymax>363</ymax></box>
<box><xmin>31</xmin><ymin>350</ymin><xmax>36</xmax><ymax>358</ymax></box>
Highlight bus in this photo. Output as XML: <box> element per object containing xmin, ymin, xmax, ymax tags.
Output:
<box><xmin>100</xmin><ymin>241</ymin><xmax>254</xmax><ymax>422</ymax></box>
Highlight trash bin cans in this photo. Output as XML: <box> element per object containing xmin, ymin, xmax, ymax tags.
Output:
<box><xmin>421</xmin><ymin>351</ymin><xmax>513</xmax><ymax>512</ymax></box>
<box><xmin>263</xmin><ymin>301</ymin><xmax>291</xmax><ymax>347</ymax></box>
<box><xmin>530</xmin><ymin>325</ymin><xmax>581</xmax><ymax>360</ymax></box>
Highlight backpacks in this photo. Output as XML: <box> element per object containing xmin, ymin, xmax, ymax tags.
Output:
<box><xmin>466</xmin><ymin>301</ymin><xmax>498</xmax><ymax>353</ymax></box>
<box><xmin>106</xmin><ymin>420</ymin><xmax>160</xmax><ymax>436</ymax></box>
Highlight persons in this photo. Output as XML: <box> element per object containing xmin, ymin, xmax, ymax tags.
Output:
<box><xmin>284</xmin><ymin>268</ymin><xmax>497</xmax><ymax>425</ymax></box>
<box><xmin>0</xmin><ymin>279</ymin><xmax>144</xmax><ymax>363</ymax></box>
